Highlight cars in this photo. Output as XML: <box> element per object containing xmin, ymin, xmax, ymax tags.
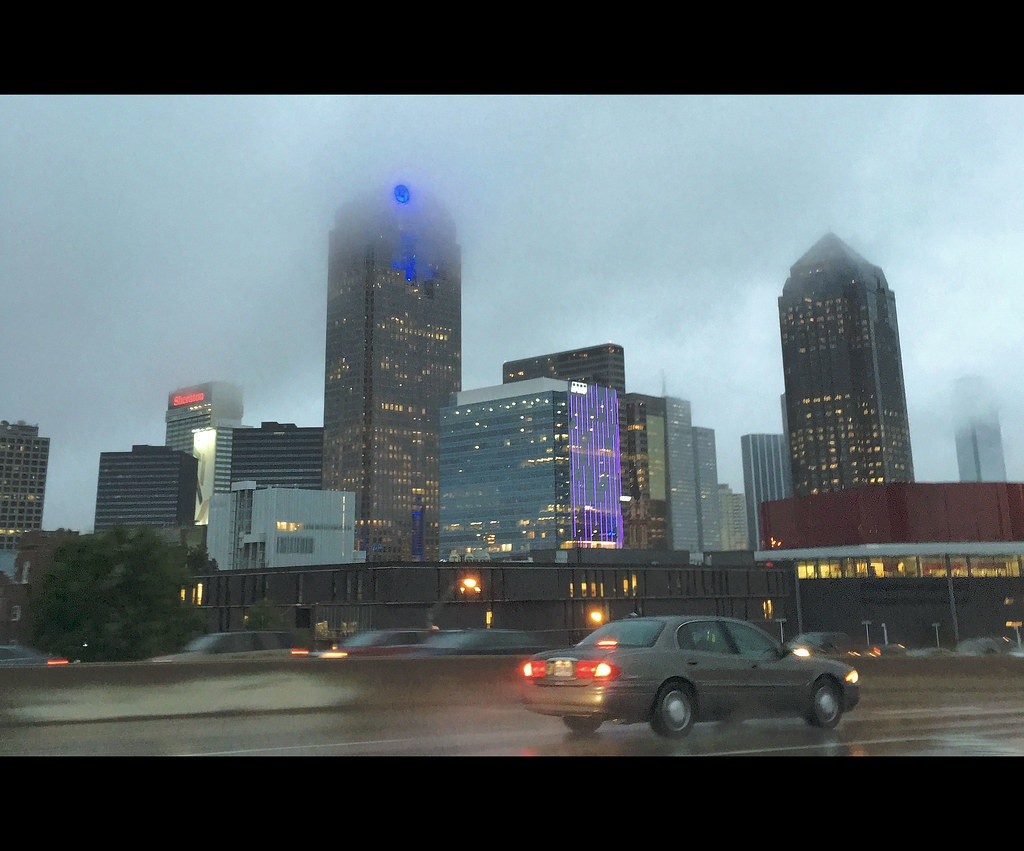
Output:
<box><xmin>519</xmin><ymin>616</ymin><xmax>860</xmax><ymax>739</ymax></box>
<box><xmin>787</xmin><ymin>633</ymin><xmax>1024</xmax><ymax>659</ymax></box>
<box><xmin>148</xmin><ymin>629</ymin><xmax>573</xmax><ymax>662</ymax></box>
<box><xmin>0</xmin><ymin>646</ymin><xmax>69</xmax><ymax>665</ymax></box>
